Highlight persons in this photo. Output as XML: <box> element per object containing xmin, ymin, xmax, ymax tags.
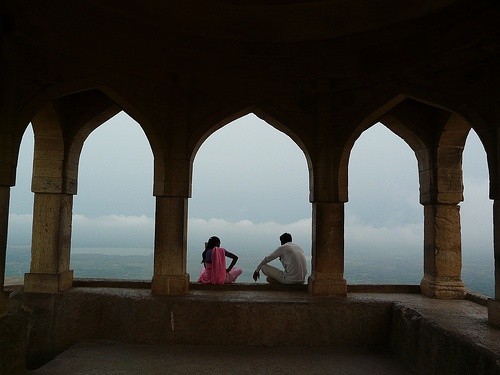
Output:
<box><xmin>253</xmin><ymin>233</ymin><xmax>308</xmax><ymax>284</ymax></box>
<box><xmin>197</xmin><ymin>235</ymin><xmax>242</xmax><ymax>285</ymax></box>
